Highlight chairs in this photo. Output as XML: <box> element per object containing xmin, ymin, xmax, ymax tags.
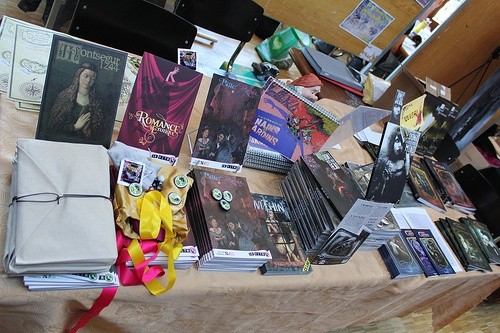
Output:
<box><xmin>65</xmin><ymin>0</ymin><xmax>265</xmax><ymax>78</ymax></box>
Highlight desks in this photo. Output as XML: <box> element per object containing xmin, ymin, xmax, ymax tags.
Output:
<box><xmin>0</xmin><ymin>37</ymin><xmax>500</xmax><ymax>333</ymax></box>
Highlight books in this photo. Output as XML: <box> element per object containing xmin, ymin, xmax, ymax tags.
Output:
<box><xmin>458</xmin><ymin>217</ymin><xmax>500</xmax><ymax>264</ymax></box>
<box><xmin>250</xmin><ymin>192</ymin><xmax>314</xmax><ymax>275</ymax></box>
<box><xmin>125</xmin><ymin>204</ymin><xmax>200</xmax><ymax>270</ymax></box>
<box><xmin>190</xmin><ymin>73</ymin><xmax>262</xmax><ymax>173</ymax></box>
<box><xmin>24</xmin><ymin>265</ymin><xmax>119</xmax><ymax>291</ymax></box>
<box><xmin>423</xmin><ymin>157</ymin><xmax>476</xmax><ymax>215</ymax></box>
<box><xmin>243</xmin><ymin>76</ymin><xmax>341</xmax><ymax>175</ymax></box>
<box><xmin>406</xmin><ymin>160</ymin><xmax>447</xmax><ymax>214</ymax></box>
<box><xmin>117</xmin><ymin>52</ymin><xmax>204</xmax><ymax>167</ymax></box>
<box><xmin>435</xmin><ymin>216</ymin><xmax>493</xmax><ymax>273</ymax></box>
<box><xmin>184</xmin><ymin>167</ymin><xmax>273</xmax><ymax>273</ymax></box>
<box><xmin>279</xmin><ymin>151</ymin><xmax>402</xmax><ymax>252</ymax></box>
<box><xmin>35</xmin><ymin>34</ymin><xmax>128</xmax><ymax>150</ymax></box>
<box><xmin>301</xmin><ymin>46</ymin><xmax>363</xmax><ymax>92</ymax></box>
<box><xmin>378</xmin><ymin>229</ymin><xmax>457</xmax><ymax>280</ymax></box>
<box><xmin>363</xmin><ymin>122</ymin><xmax>422</xmax><ymax>204</ymax></box>
